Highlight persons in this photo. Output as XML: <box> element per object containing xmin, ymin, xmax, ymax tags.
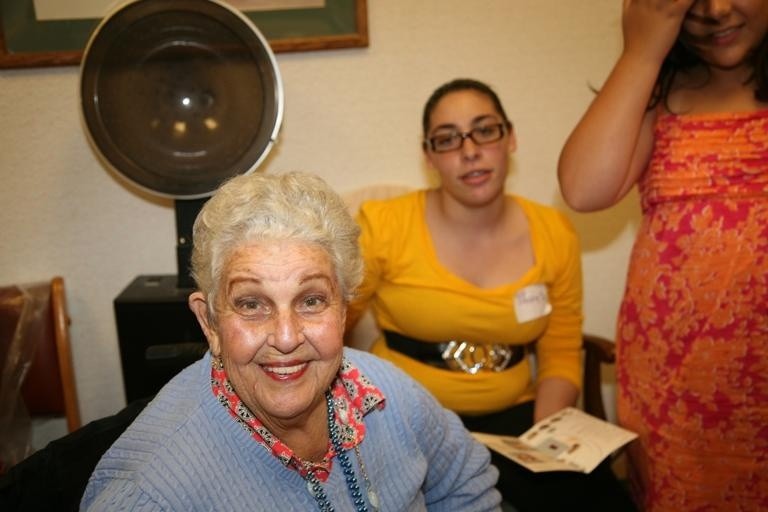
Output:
<box><xmin>79</xmin><ymin>173</ymin><xmax>503</xmax><ymax>512</ymax></box>
<box><xmin>342</xmin><ymin>79</ymin><xmax>612</xmax><ymax>511</ymax></box>
<box><xmin>557</xmin><ymin>1</ymin><xmax>768</xmax><ymax>512</ymax></box>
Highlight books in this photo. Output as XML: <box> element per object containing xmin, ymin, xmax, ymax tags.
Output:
<box><xmin>469</xmin><ymin>406</ymin><xmax>640</xmax><ymax>474</ymax></box>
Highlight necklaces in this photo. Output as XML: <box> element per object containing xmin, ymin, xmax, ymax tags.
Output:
<box><xmin>277</xmin><ymin>390</ymin><xmax>378</xmax><ymax>512</ymax></box>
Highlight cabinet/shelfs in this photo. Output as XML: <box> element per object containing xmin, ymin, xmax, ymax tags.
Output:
<box><xmin>113</xmin><ymin>274</ymin><xmax>210</xmax><ymax>405</ymax></box>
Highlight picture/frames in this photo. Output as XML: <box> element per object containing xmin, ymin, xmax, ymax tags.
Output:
<box><xmin>0</xmin><ymin>0</ymin><xmax>369</xmax><ymax>70</ymax></box>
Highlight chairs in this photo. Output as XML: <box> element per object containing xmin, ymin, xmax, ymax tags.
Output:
<box><xmin>1</xmin><ymin>275</ymin><xmax>81</xmax><ymax>469</ymax></box>
<box><xmin>583</xmin><ymin>332</ymin><xmax>617</xmax><ymax>424</ymax></box>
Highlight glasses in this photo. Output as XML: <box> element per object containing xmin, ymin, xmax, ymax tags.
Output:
<box><xmin>431</xmin><ymin>124</ymin><xmax>504</xmax><ymax>153</ymax></box>
